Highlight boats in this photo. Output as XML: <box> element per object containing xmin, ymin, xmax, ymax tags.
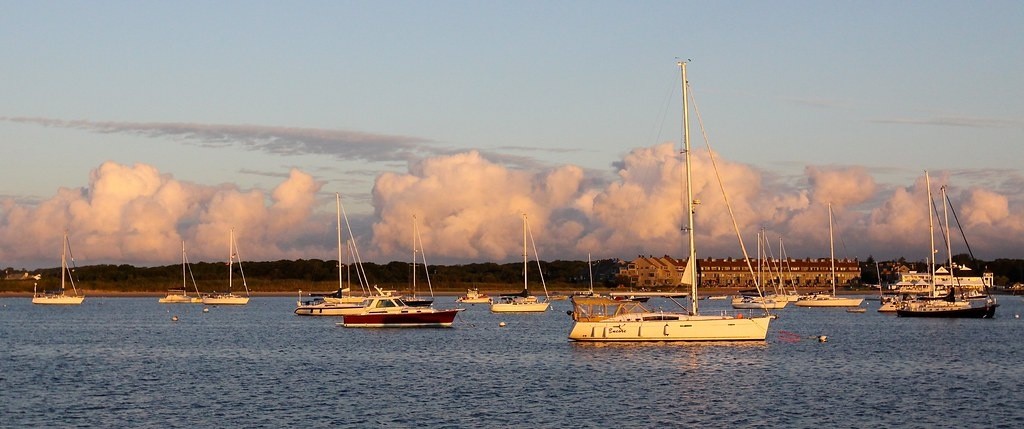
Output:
<box><xmin>454</xmin><ymin>288</ymin><xmax>494</xmax><ymax>304</ymax></box>
<box><xmin>335</xmin><ymin>295</ymin><xmax>466</xmax><ymax>329</ymax></box>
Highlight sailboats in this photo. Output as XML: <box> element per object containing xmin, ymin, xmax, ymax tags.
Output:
<box><xmin>292</xmin><ymin>192</ymin><xmax>440</xmax><ymax>316</ymax></box>
<box><xmin>566</xmin><ymin>60</ymin><xmax>778</xmax><ymax>342</ymax></box>
<box><xmin>489</xmin><ymin>211</ymin><xmax>551</xmax><ymax>312</ymax></box>
<box><xmin>30</xmin><ymin>234</ymin><xmax>86</xmax><ymax>305</ymax></box>
<box><xmin>546</xmin><ymin>168</ymin><xmax>998</xmax><ymax>319</ymax></box>
<box><xmin>159</xmin><ymin>241</ymin><xmax>206</xmax><ymax>305</ymax></box>
<box><xmin>202</xmin><ymin>227</ymin><xmax>251</xmax><ymax>306</ymax></box>
